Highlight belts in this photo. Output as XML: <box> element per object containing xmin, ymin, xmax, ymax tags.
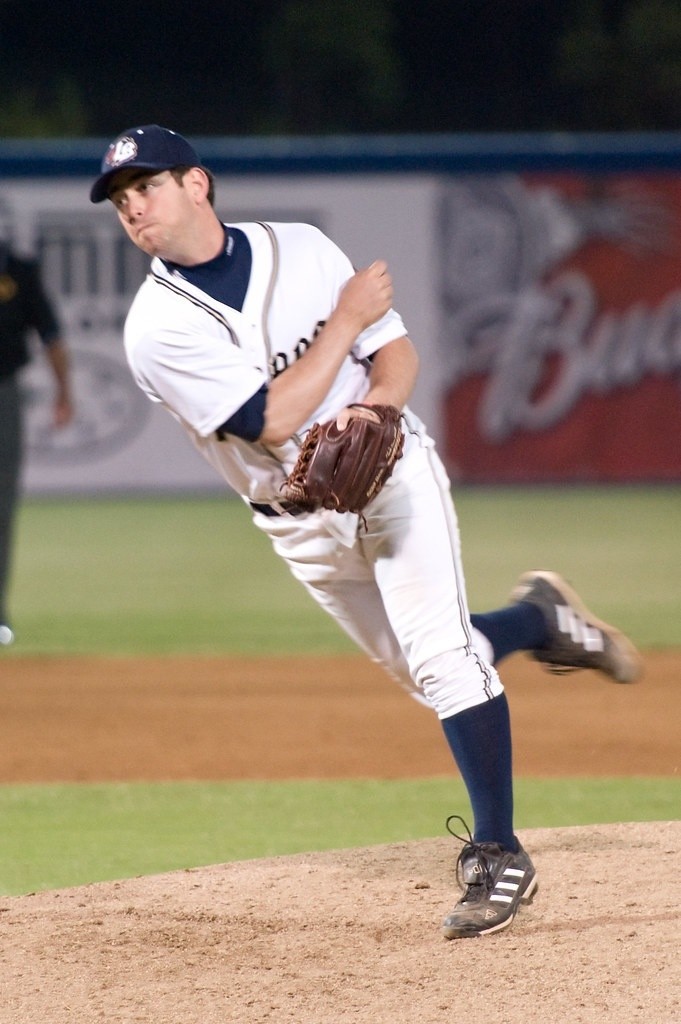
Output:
<box><xmin>249</xmin><ymin>501</ymin><xmax>306</xmax><ymax>517</ymax></box>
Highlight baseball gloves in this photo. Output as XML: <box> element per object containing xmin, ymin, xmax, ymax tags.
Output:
<box><xmin>277</xmin><ymin>402</ymin><xmax>405</xmax><ymax>533</ymax></box>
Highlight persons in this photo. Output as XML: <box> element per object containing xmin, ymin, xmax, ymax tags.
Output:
<box><xmin>90</xmin><ymin>123</ymin><xmax>645</xmax><ymax>942</ymax></box>
<box><xmin>0</xmin><ymin>194</ymin><xmax>75</xmax><ymax>644</ymax></box>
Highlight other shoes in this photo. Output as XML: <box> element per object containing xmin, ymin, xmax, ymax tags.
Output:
<box><xmin>0</xmin><ymin>625</ymin><xmax>14</xmax><ymax>646</ymax></box>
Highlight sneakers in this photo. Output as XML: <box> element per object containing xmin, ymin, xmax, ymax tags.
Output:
<box><xmin>508</xmin><ymin>568</ymin><xmax>645</xmax><ymax>683</ymax></box>
<box><xmin>441</xmin><ymin>815</ymin><xmax>537</xmax><ymax>940</ymax></box>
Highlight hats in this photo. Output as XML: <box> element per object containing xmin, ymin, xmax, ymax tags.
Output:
<box><xmin>90</xmin><ymin>123</ymin><xmax>201</xmax><ymax>204</ymax></box>
